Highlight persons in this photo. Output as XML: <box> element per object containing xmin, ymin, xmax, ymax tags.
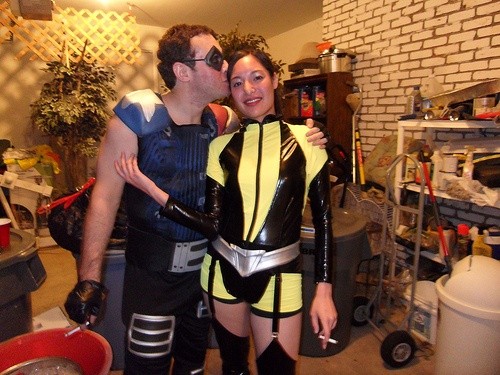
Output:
<box><xmin>114</xmin><ymin>46</ymin><xmax>338</xmax><ymax>375</ymax></box>
<box><xmin>64</xmin><ymin>23</ymin><xmax>327</xmax><ymax>375</ymax></box>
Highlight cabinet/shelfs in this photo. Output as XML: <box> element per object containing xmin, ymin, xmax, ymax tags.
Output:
<box><xmin>388</xmin><ymin>116</ymin><xmax>500</xmax><ymax>354</ymax></box>
<box><xmin>273</xmin><ymin>70</ymin><xmax>356</xmax><ymax>200</ymax></box>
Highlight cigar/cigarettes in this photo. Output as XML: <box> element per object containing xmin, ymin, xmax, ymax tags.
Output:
<box><xmin>65</xmin><ymin>321</ymin><xmax>89</xmax><ymax>337</ymax></box>
<box><xmin>318</xmin><ymin>336</ymin><xmax>338</xmax><ymax>344</ymax></box>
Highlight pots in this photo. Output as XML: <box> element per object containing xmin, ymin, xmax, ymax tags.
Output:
<box><xmin>317</xmin><ymin>47</ymin><xmax>356</xmax><ymax>74</ymax></box>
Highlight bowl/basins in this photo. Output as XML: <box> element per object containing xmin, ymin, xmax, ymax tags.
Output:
<box><xmin>0</xmin><ymin>327</ymin><xmax>113</xmax><ymax>375</ymax></box>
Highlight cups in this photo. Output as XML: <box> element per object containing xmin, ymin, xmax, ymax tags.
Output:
<box><xmin>0</xmin><ymin>218</ymin><xmax>12</xmax><ymax>249</ymax></box>
<box><xmin>416</xmin><ymin>161</ymin><xmax>431</xmax><ymax>184</ymax></box>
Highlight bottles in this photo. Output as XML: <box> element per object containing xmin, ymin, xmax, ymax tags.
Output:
<box><xmin>406</xmin><ymin>86</ymin><xmax>423</xmax><ymax>119</ymax></box>
<box><xmin>439</xmin><ymin>222</ymin><xmax>479</xmax><ymax>261</ymax></box>
<box><xmin>420</xmin><ymin>75</ymin><xmax>444</xmax><ymax>112</ymax></box>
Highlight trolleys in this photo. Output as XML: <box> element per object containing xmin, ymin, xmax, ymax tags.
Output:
<box><xmin>351</xmin><ymin>153</ymin><xmax>425</xmax><ymax>368</ymax></box>
